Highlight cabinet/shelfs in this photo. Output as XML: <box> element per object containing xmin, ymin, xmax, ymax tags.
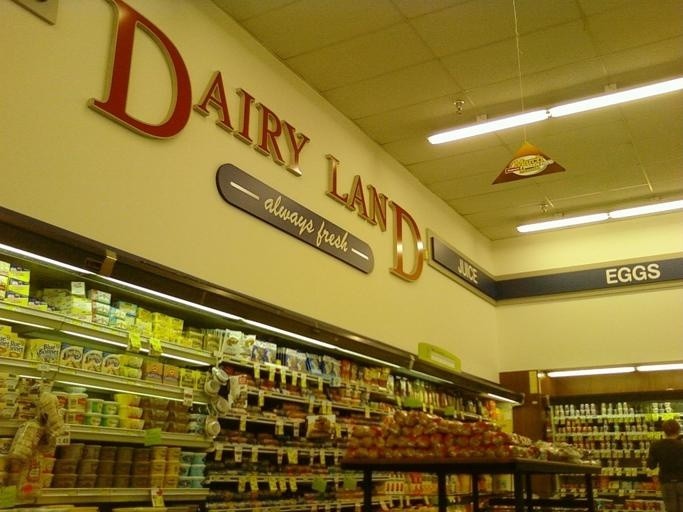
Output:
<box><xmin>0</xmin><ymin>205</ymin><xmax>523</xmax><ymax>512</ymax></box>
<box><xmin>543</xmin><ymin>390</ymin><xmax>683</xmax><ymax>512</ymax></box>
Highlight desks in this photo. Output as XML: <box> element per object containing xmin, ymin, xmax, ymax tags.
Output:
<box><xmin>340</xmin><ymin>457</ymin><xmax>601</xmax><ymax>512</ymax></box>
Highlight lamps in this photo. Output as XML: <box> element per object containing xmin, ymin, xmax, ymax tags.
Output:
<box><xmin>512</xmin><ymin>196</ymin><xmax>683</xmax><ymax>233</ymax></box>
<box><xmin>426</xmin><ymin>76</ymin><xmax>683</xmax><ymax>145</ymax></box>
<box><xmin>493</xmin><ymin>0</ymin><xmax>565</xmax><ymax>184</ymax></box>
